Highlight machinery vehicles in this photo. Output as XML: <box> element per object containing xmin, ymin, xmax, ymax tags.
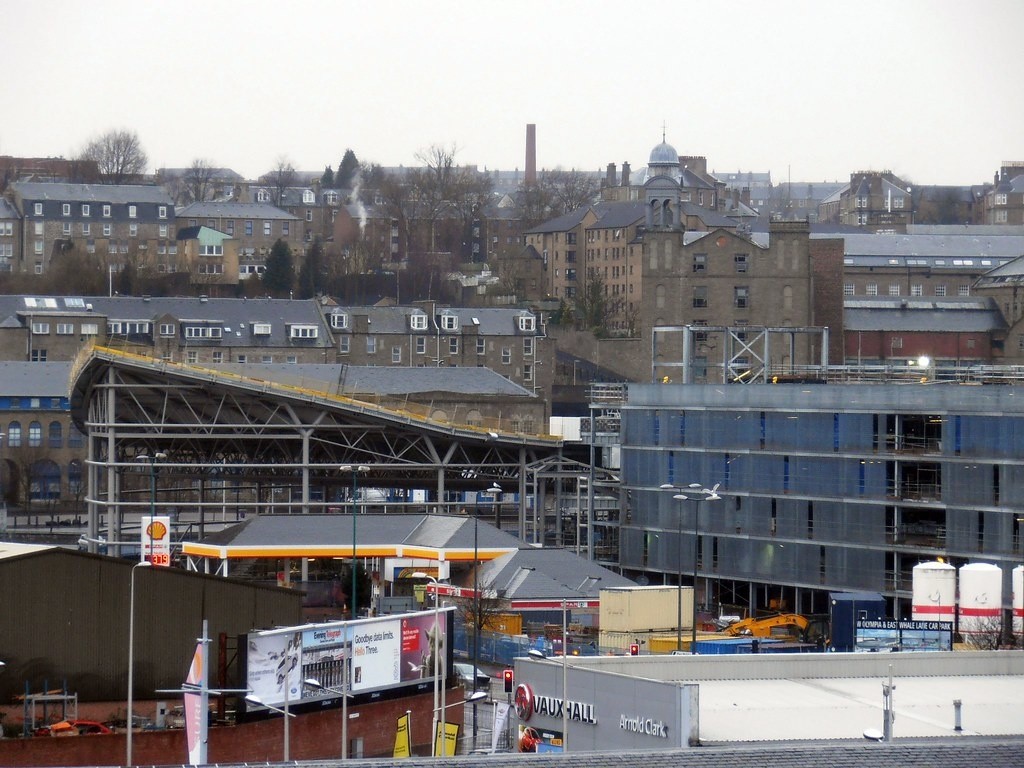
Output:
<box><xmin>717</xmin><ymin>614</ymin><xmax>830</xmax><ymax>653</ymax></box>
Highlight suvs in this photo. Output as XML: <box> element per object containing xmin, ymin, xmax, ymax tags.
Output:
<box><xmin>547</xmin><ymin>643</ymin><xmax>596</xmax><ymax>656</ymax></box>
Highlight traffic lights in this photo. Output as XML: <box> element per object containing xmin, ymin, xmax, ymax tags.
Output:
<box><xmin>630</xmin><ymin>644</ymin><xmax>639</xmax><ymax>656</ymax></box>
<box><xmin>504</xmin><ymin>670</ymin><xmax>513</xmax><ymax>693</ymax></box>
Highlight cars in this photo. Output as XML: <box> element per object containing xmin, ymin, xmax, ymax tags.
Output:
<box><xmin>77</xmin><ymin>534</ymin><xmax>106</xmax><ymax>553</ymax></box>
<box><xmin>453</xmin><ymin>663</ymin><xmax>492</xmax><ymax>691</ymax></box>
<box><xmin>35</xmin><ymin>720</ymin><xmax>111</xmax><ymax>736</ymax></box>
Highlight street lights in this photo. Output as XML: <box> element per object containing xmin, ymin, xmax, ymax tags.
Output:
<box><xmin>244</xmin><ymin>636</ymin><xmax>296</xmax><ymax>763</ymax></box>
<box><xmin>340</xmin><ymin>465</ymin><xmax>371</xmax><ymax>620</ymax></box>
<box><xmin>661</xmin><ymin>483</ymin><xmax>702</xmax><ymax>646</ymax></box>
<box><xmin>303</xmin><ymin>623</ymin><xmax>355</xmax><ymax>759</ymax></box>
<box><xmin>136</xmin><ymin>453</ymin><xmax>166</xmax><ymax>554</ymax></box>
<box><xmin>412</xmin><ymin>572</ymin><xmax>487</xmax><ymax>756</ymax></box>
<box><xmin>127</xmin><ymin>561</ymin><xmax>152</xmax><ymax>768</ymax></box>
<box><xmin>528</xmin><ymin>598</ymin><xmax>575</xmax><ymax>754</ymax></box>
<box><xmin>674</xmin><ymin>495</ymin><xmax>721</xmax><ymax>655</ymax></box>
<box><xmin>473</xmin><ymin>488</ymin><xmax>502</xmax><ymax>748</ymax></box>
